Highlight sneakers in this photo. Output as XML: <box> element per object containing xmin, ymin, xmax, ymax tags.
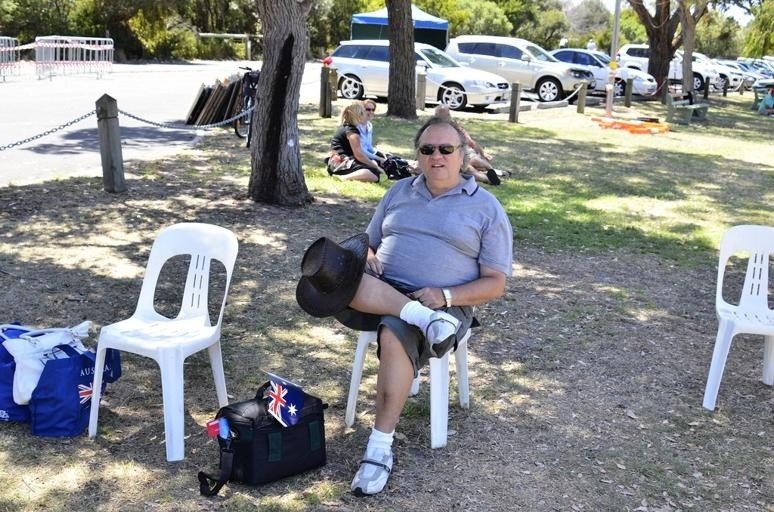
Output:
<box><xmin>424</xmin><ymin>309</ymin><xmax>462</xmax><ymax>358</ymax></box>
<box><xmin>350</xmin><ymin>445</ymin><xmax>394</xmax><ymax>496</ymax></box>
<box><xmin>486</xmin><ymin>169</ymin><xmax>511</xmax><ymax>186</ymax></box>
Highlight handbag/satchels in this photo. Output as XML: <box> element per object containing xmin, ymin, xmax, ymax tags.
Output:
<box><xmin>0</xmin><ymin>323</ymin><xmax>45</xmax><ymax>422</ymax></box>
<box><xmin>27</xmin><ymin>342</ymin><xmax>122</xmax><ymax>439</ymax></box>
<box><xmin>213</xmin><ymin>379</ymin><xmax>328</xmax><ymax>485</ymax></box>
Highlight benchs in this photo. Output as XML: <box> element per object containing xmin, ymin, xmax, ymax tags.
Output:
<box><xmin>750</xmin><ymin>85</ymin><xmax>768</xmax><ymax>110</ymax></box>
<box><xmin>661</xmin><ymin>87</ymin><xmax>709</xmax><ymax>126</ymax></box>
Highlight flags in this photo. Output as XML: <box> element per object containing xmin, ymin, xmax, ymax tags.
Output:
<box><xmin>265</xmin><ymin>378</ymin><xmax>301</xmax><ymax>426</ymax></box>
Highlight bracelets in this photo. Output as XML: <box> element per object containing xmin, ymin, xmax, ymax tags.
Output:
<box><xmin>441</xmin><ymin>288</ymin><xmax>453</xmax><ymax>309</ymax></box>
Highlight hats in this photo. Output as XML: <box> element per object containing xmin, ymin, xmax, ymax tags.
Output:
<box><xmin>295</xmin><ymin>233</ymin><xmax>370</xmax><ymax>318</ymax></box>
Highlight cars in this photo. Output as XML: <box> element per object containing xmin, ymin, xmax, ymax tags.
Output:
<box><xmin>612</xmin><ymin>42</ymin><xmax>774</xmax><ymax>94</ymax></box>
<box><xmin>322</xmin><ymin>36</ymin><xmax>516</xmax><ymax>111</ymax></box>
<box><xmin>535</xmin><ymin>42</ymin><xmax>661</xmax><ymax>103</ymax></box>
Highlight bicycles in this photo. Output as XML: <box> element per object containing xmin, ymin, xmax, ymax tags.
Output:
<box><xmin>230</xmin><ymin>63</ymin><xmax>259</xmax><ymax>150</ymax></box>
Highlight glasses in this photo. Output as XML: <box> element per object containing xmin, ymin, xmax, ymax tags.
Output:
<box><xmin>419</xmin><ymin>143</ymin><xmax>462</xmax><ymax>155</ymax></box>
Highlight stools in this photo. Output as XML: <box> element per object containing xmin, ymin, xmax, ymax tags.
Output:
<box><xmin>344</xmin><ymin>321</ymin><xmax>474</xmax><ymax>450</ymax></box>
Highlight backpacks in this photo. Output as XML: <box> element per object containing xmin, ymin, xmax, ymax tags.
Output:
<box><xmin>383</xmin><ymin>155</ymin><xmax>412</xmax><ymax>180</ymax></box>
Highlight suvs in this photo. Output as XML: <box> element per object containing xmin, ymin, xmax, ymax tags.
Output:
<box><xmin>438</xmin><ymin>32</ymin><xmax>596</xmax><ymax>102</ymax></box>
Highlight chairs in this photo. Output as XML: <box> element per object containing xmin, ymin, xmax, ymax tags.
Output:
<box><xmin>698</xmin><ymin>222</ymin><xmax>773</xmax><ymax>412</ymax></box>
<box><xmin>84</xmin><ymin>220</ymin><xmax>240</xmax><ymax>464</ymax></box>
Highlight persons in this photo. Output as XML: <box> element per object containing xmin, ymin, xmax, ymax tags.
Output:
<box><xmin>758</xmin><ymin>88</ymin><xmax>774</xmax><ymax>117</ymax></box>
<box><xmin>558</xmin><ymin>36</ymin><xmax>568</xmax><ymax>48</ymax></box>
<box><xmin>586</xmin><ymin>39</ymin><xmax>595</xmax><ymax>51</ymax></box>
<box><xmin>433</xmin><ymin>104</ymin><xmax>511</xmax><ymax>187</ymax></box>
<box><xmin>347</xmin><ymin>118</ymin><xmax>514</xmax><ymax>495</ymax></box>
<box><xmin>329</xmin><ymin>100</ymin><xmax>384</xmax><ymax>183</ymax></box>
<box><xmin>358</xmin><ymin>98</ymin><xmax>422</xmax><ymax>176</ymax></box>
